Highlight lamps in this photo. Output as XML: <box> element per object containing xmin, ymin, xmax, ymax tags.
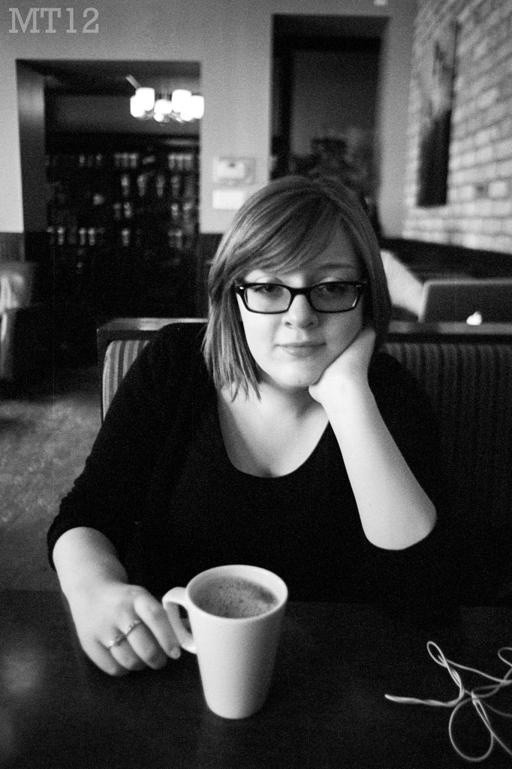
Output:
<box><xmin>127</xmin><ymin>79</ymin><xmax>203</xmax><ymax>125</ymax></box>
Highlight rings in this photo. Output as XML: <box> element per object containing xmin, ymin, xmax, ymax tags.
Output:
<box><xmin>105</xmin><ymin>635</ymin><xmax>127</xmax><ymax>650</ymax></box>
<box><xmin>122</xmin><ymin>620</ymin><xmax>142</xmax><ymax>638</ymax></box>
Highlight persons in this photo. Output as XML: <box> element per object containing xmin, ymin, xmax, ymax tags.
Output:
<box><xmin>44</xmin><ymin>174</ymin><xmax>454</xmax><ymax>679</ymax></box>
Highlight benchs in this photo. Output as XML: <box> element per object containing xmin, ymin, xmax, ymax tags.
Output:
<box><xmin>83</xmin><ymin>315</ymin><xmax>508</xmax><ymax>577</ymax></box>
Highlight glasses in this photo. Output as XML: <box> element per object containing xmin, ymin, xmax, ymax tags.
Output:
<box><xmin>236</xmin><ymin>275</ymin><xmax>366</xmax><ymax>315</ymax></box>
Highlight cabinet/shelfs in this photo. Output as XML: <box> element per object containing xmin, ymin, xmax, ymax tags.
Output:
<box><xmin>39</xmin><ymin>118</ymin><xmax>200</xmax><ymax>308</ymax></box>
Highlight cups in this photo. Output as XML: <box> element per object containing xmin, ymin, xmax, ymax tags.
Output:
<box><xmin>161</xmin><ymin>563</ymin><xmax>288</xmax><ymax>722</ymax></box>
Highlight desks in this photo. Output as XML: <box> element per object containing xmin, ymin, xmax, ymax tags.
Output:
<box><xmin>0</xmin><ymin>585</ymin><xmax>512</xmax><ymax>767</ymax></box>
